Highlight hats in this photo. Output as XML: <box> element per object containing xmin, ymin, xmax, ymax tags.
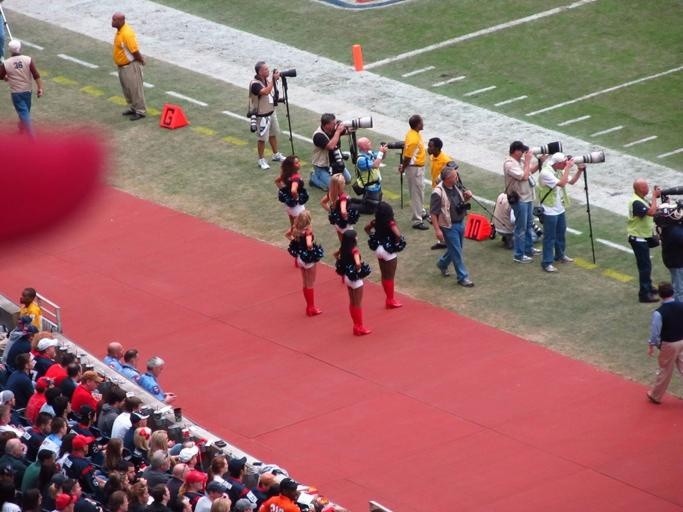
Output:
<box><xmin>548</xmin><ymin>152</ymin><xmax>568</xmax><ymax>166</ymax></box>
<box><xmin>1</xmin><ymin>314</ymin><xmax>301</xmax><ymax>511</ymax></box>
<box><xmin>229</xmin><ymin>456</ymin><xmax>247</xmax><ymax>473</ymax></box>
<box><xmin>1</xmin><ymin>463</ymin><xmax>17</xmax><ymax>476</ymax></box>
<box><xmin>235</xmin><ymin>498</ymin><xmax>257</xmax><ymax>511</ymax></box>
<box><xmin>73</xmin><ymin>434</ymin><xmax>93</xmax><ymax>450</ymax></box>
<box><xmin>8</xmin><ymin>39</ymin><xmax>22</xmax><ymax>52</ymax></box>
<box><xmin>130</xmin><ymin>410</ymin><xmax>150</xmax><ymax>424</ymax></box>
<box><xmin>185</xmin><ymin>470</ymin><xmax>206</xmax><ymax>483</ymax></box>
<box><xmin>36</xmin><ymin>376</ymin><xmax>55</xmax><ymax>391</ymax></box>
<box><xmin>22</xmin><ymin>325</ymin><xmax>38</xmax><ymax>336</ymax></box>
<box><xmin>82</xmin><ymin>371</ymin><xmax>104</xmax><ymax>382</ymax></box>
<box><xmin>179</xmin><ymin>446</ymin><xmax>198</xmax><ymax>463</ymax></box>
<box><xmin>280</xmin><ymin>478</ymin><xmax>300</xmax><ymax>490</ymax></box>
<box><xmin>51</xmin><ymin>474</ymin><xmax>65</xmax><ymax>487</ymax></box>
<box><xmin>37</xmin><ymin>338</ymin><xmax>58</xmax><ymax>351</ymax></box>
<box><xmin>206</xmin><ymin>481</ymin><xmax>227</xmax><ymax>493</ymax></box>
<box><xmin>510</xmin><ymin>141</ymin><xmax>529</xmax><ymax>152</ymax></box>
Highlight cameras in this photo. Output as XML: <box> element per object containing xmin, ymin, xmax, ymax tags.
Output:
<box><xmin>507</xmin><ymin>192</ymin><xmax>519</xmax><ymax>204</ymax></box>
<box><xmin>456</xmin><ymin>200</ymin><xmax>471</xmax><ymax>213</ymax></box>
<box><xmin>533</xmin><ymin>207</ymin><xmax>544</xmax><ymax>224</ymax></box>
<box><xmin>273</xmin><ymin>95</ymin><xmax>286</xmax><ymax>106</ymax></box>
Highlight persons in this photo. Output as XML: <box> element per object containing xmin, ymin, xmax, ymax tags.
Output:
<box><xmin>363</xmin><ymin>202</ymin><xmax>404</xmax><ymax>309</ymax></box>
<box><xmin>0</xmin><ymin>37</ymin><xmax>45</xmax><ymax>142</ymax></box>
<box><xmin>426</xmin><ymin>137</ymin><xmax>455</xmax><ymax>251</ymax></box>
<box><xmin>627</xmin><ymin>177</ymin><xmax>660</xmax><ymax>303</ymax></box>
<box><xmin>346</xmin><ymin>137</ymin><xmax>388</xmax><ymax>214</ymax></box>
<box><xmin>645</xmin><ymin>281</ymin><xmax>682</xmax><ymax>407</ymax></box>
<box><xmin>10</xmin><ymin>288</ymin><xmax>41</xmax><ymax>329</ymax></box>
<box><xmin>308</xmin><ymin>113</ymin><xmax>351</xmax><ymax>191</ymax></box>
<box><xmin>398</xmin><ymin>115</ymin><xmax>431</xmax><ymax>230</ymax></box>
<box><xmin>502</xmin><ymin>140</ymin><xmax>548</xmax><ymax>265</ymax></box>
<box><xmin>319</xmin><ymin>173</ymin><xmax>353</xmax><ymax>262</ymax></box>
<box><xmin>538</xmin><ymin>152</ymin><xmax>586</xmax><ymax>273</ymax></box>
<box><xmin>492</xmin><ymin>191</ymin><xmax>538</xmax><ymax>249</ymax></box>
<box><xmin>284</xmin><ymin>210</ymin><xmax>323</xmax><ymax>317</ymax></box>
<box><xmin>660</xmin><ymin>197</ymin><xmax>682</xmax><ymax>304</ymax></box>
<box><xmin>247</xmin><ymin>61</ymin><xmax>288</xmax><ymax>171</ymax></box>
<box><xmin>331</xmin><ymin>230</ymin><xmax>373</xmax><ymax>336</ymax></box>
<box><xmin>274</xmin><ymin>155</ymin><xmax>306</xmax><ymax>240</ymax></box>
<box><xmin>109</xmin><ymin>11</ymin><xmax>151</xmax><ymax>122</ymax></box>
<box><xmin>429</xmin><ymin>167</ymin><xmax>477</xmax><ymax>287</ymax></box>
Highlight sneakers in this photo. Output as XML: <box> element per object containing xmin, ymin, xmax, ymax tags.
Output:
<box><xmin>544</xmin><ymin>264</ymin><xmax>559</xmax><ymax>273</ymax></box>
<box><xmin>412</xmin><ymin>211</ymin><xmax>430</xmax><ymax>230</ymax></box>
<box><xmin>457</xmin><ymin>278</ymin><xmax>474</xmax><ymax>288</ymax></box>
<box><xmin>122</xmin><ymin>108</ymin><xmax>145</xmax><ymax>121</ymax></box>
<box><xmin>430</xmin><ymin>242</ymin><xmax>447</xmax><ymax>250</ymax></box>
<box><xmin>639</xmin><ymin>285</ymin><xmax>662</xmax><ymax>303</ymax></box>
<box><xmin>559</xmin><ymin>255</ymin><xmax>576</xmax><ymax>264</ymax></box>
<box><xmin>436</xmin><ymin>261</ymin><xmax>450</xmax><ymax>277</ymax></box>
<box><xmin>525</xmin><ymin>248</ymin><xmax>542</xmax><ymax>256</ymax></box>
<box><xmin>646</xmin><ymin>389</ymin><xmax>661</xmax><ymax>406</ymax></box>
<box><xmin>257</xmin><ymin>149</ymin><xmax>288</xmax><ymax>168</ymax></box>
<box><xmin>512</xmin><ymin>254</ymin><xmax>534</xmax><ymax>265</ymax></box>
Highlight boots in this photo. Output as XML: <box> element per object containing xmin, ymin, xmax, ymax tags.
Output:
<box><xmin>348</xmin><ymin>304</ymin><xmax>373</xmax><ymax>336</ymax></box>
<box><xmin>381</xmin><ymin>277</ymin><xmax>403</xmax><ymax>310</ymax></box>
<box><xmin>302</xmin><ymin>287</ymin><xmax>323</xmax><ymax>317</ymax></box>
